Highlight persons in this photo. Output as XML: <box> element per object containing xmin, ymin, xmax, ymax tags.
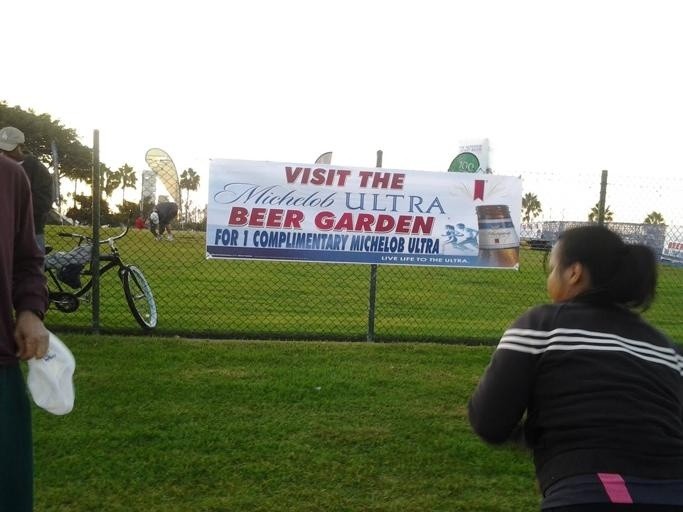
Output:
<box><xmin>0</xmin><ymin>147</ymin><xmax>55</xmax><ymax>511</ymax></box>
<box><xmin>154</xmin><ymin>194</ymin><xmax>179</xmax><ymax>242</ymax></box>
<box><xmin>134</xmin><ymin>214</ymin><xmax>147</xmax><ymax>230</ymax></box>
<box><xmin>1</xmin><ymin>123</ymin><xmax>55</xmax><ymax>270</ymax></box>
<box><xmin>464</xmin><ymin>221</ymin><xmax>681</xmax><ymax>512</ymax></box>
<box><xmin>147</xmin><ymin>208</ymin><xmax>162</xmax><ymax>241</ymax></box>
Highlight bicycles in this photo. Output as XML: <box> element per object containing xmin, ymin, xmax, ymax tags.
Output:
<box><xmin>45</xmin><ymin>211</ymin><xmax>158</xmax><ymax>329</ymax></box>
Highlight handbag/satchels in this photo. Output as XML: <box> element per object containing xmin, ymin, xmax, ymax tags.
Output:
<box><xmin>151</xmin><ymin>212</ymin><xmax>159</xmax><ymax>224</ymax></box>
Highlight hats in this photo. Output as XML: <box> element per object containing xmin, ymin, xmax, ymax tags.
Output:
<box><xmin>0</xmin><ymin>127</ymin><xmax>25</xmax><ymax>152</ymax></box>
<box><xmin>26</xmin><ymin>330</ymin><xmax>76</xmax><ymax>416</ymax></box>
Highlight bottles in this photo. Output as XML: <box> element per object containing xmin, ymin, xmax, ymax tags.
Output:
<box><xmin>476</xmin><ymin>204</ymin><xmax>519</xmax><ymax>267</ymax></box>
